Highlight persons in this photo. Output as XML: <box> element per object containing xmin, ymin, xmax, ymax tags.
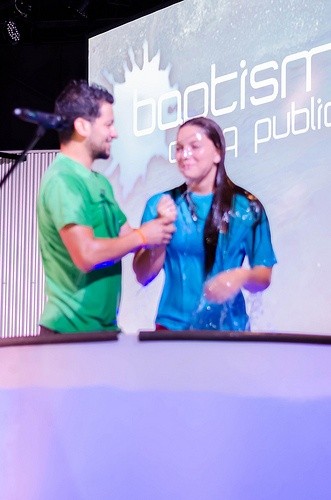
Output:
<box><xmin>34</xmin><ymin>83</ymin><xmax>175</xmax><ymax>334</ymax></box>
<box><xmin>133</xmin><ymin>118</ymin><xmax>275</xmax><ymax>334</ymax></box>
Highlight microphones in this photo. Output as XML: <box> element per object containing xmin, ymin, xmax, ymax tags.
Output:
<box><xmin>15</xmin><ymin>109</ymin><xmax>74</xmax><ymax>134</ymax></box>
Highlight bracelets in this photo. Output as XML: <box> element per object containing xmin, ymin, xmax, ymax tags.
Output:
<box><xmin>134</xmin><ymin>228</ymin><xmax>147</xmax><ymax>247</ymax></box>
